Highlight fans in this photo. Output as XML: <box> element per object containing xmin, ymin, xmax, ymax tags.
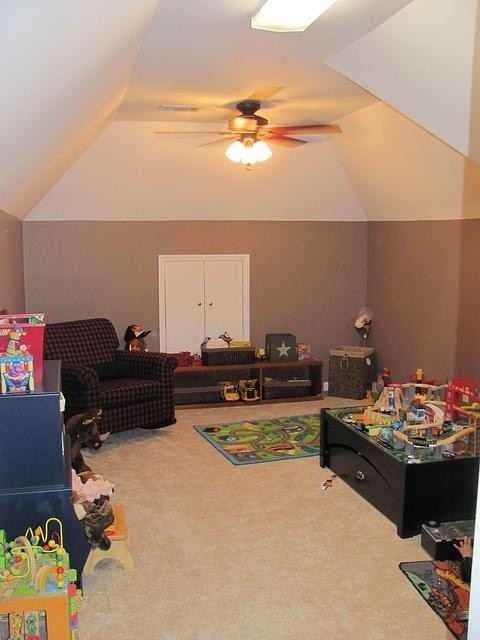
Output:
<box><xmin>156</xmin><ymin>101</ymin><xmax>344</xmax><ymax>148</ymax></box>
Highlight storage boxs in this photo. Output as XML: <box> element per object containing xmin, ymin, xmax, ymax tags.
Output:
<box><xmin>266</xmin><ymin>333</ymin><xmax>296</xmax><ymax>362</ymax></box>
<box><xmin>0</xmin><ymin>312</ymin><xmax>47</xmax><ymax>384</ymax></box>
<box><xmin>262</xmin><ymin>380</ymin><xmax>312</xmax><ymax>400</ymax></box>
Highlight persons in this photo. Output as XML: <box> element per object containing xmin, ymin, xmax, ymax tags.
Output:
<box><xmin>453</xmin><ymin>536</ymin><xmax>473</xmax><ymax>586</ymax></box>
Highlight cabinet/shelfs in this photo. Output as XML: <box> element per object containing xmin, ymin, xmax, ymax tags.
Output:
<box><xmin>0</xmin><ymin>359</ymin><xmax>91</xmax><ymax>598</ymax></box>
<box><xmin>172</xmin><ymin>358</ymin><xmax>324</xmax><ymax>410</ymax></box>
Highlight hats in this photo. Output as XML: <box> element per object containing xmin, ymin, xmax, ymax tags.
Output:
<box><xmin>124</xmin><ymin>325</ymin><xmax>151</xmax><ymax>341</ymax></box>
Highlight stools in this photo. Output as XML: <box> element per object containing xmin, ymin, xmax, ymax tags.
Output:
<box><xmin>81</xmin><ymin>503</ymin><xmax>133</xmax><ymax>576</ymax></box>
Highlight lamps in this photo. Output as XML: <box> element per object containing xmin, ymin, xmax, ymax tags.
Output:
<box><xmin>227</xmin><ymin>136</ymin><xmax>271</xmax><ymax>172</ymax></box>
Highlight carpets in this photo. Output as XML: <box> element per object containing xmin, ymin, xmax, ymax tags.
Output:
<box><xmin>398</xmin><ymin>560</ymin><xmax>470</xmax><ymax>640</ymax></box>
<box><xmin>192</xmin><ymin>413</ymin><xmax>321</xmax><ymax>466</ymax></box>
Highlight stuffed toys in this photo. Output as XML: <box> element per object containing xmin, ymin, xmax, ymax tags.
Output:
<box><xmin>72</xmin><ymin>468</ymin><xmax>114</xmax><ymax>552</ymax></box>
<box><xmin>65</xmin><ymin>408</ymin><xmax>110</xmax><ymax>485</ymax></box>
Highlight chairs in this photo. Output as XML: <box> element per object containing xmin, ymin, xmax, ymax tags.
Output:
<box><xmin>43</xmin><ymin>317</ymin><xmax>178</xmax><ymax>435</ymax></box>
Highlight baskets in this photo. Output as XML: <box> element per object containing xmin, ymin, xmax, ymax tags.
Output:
<box><xmin>262</xmin><ymin>373</ymin><xmax>312</xmax><ymax>399</ymax></box>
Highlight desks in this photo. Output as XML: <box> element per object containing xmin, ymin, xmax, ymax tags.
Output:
<box><xmin>319</xmin><ymin>405</ymin><xmax>476</xmax><ymax>539</ymax></box>
